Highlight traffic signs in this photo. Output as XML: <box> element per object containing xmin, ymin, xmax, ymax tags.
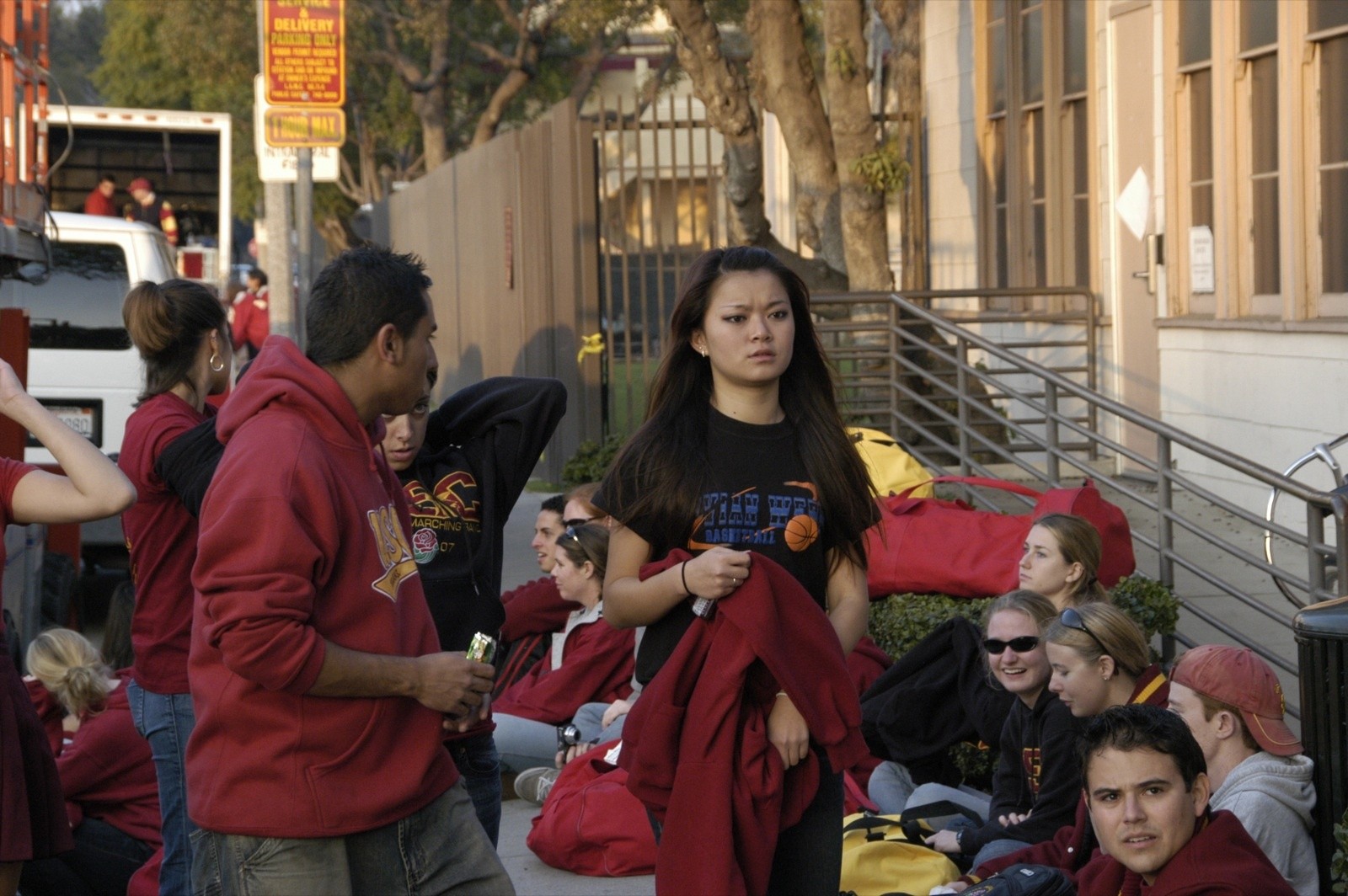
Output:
<box><xmin>263</xmin><ymin>0</ymin><xmax>350</xmax><ymax>149</ymax></box>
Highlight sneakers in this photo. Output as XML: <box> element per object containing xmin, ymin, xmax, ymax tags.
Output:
<box><xmin>513</xmin><ymin>766</ymin><xmax>561</xmax><ymax>806</ymax></box>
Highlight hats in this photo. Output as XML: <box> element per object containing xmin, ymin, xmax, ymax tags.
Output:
<box><xmin>127</xmin><ymin>177</ymin><xmax>149</xmax><ymax>190</ymax></box>
<box><xmin>1168</xmin><ymin>645</ymin><xmax>1304</xmax><ymax>757</ymax></box>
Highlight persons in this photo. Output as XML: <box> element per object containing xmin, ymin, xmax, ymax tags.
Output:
<box><xmin>923</xmin><ymin>589</ymin><xmax>1084</xmax><ymax>873</ymax></box>
<box><xmin>117</xmin><ymin>279</ymin><xmax>234</xmax><ymax>896</ymax></box>
<box><xmin>591</xmin><ymin>247</ymin><xmax>879</xmax><ymax>896</ymax></box>
<box><xmin>1164</xmin><ymin>644</ymin><xmax>1319</xmax><ymax>896</ymax></box>
<box><xmin>101</xmin><ymin>576</ymin><xmax>137</xmax><ymax>683</ymax></box>
<box><xmin>941</xmin><ymin>603</ymin><xmax>1171</xmax><ymax>896</ymax></box>
<box><xmin>183</xmin><ymin>243</ymin><xmax>516</xmax><ymax>896</ymax></box>
<box><xmin>223</xmin><ymin>266</ymin><xmax>282</xmax><ymax>387</ymax></box>
<box><xmin>928</xmin><ymin>705</ymin><xmax>1302</xmax><ymax>896</ymax></box>
<box><xmin>363</xmin><ymin>365</ymin><xmax>568</xmax><ymax>856</ymax></box>
<box><xmin>0</xmin><ymin>358</ymin><xmax>138</xmax><ymax>896</ymax></box>
<box><xmin>474</xmin><ymin>482</ymin><xmax>664</xmax><ymax>849</ymax></box>
<box><xmin>80</xmin><ymin>174</ymin><xmax>179</xmax><ymax>246</ymax></box>
<box><xmin>23</xmin><ymin>625</ymin><xmax>165</xmax><ymax>896</ymax></box>
<box><xmin>867</xmin><ymin>511</ymin><xmax>1109</xmax><ymax>824</ymax></box>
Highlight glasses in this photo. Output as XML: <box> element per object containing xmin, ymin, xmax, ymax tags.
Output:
<box><xmin>564</xmin><ymin>525</ymin><xmax>605</xmax><ymax>573</ymax></box>
<box><xmin>562</xmin><ymin>515</ymin><xmax>607</xmax><ymax>529</ymax></box>
<box><xmin>983</xmin><ymin>636</ymin><xmax>1041</xmax><ymax>654</ymax></box>
<box><xmin>1059</xmin><ymin>606</ymin><xmax>1119</xmax><ymax>675</ymax></box>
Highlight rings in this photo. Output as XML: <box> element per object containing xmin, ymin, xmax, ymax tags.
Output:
<box><xmin>731</xmin><ymin>578</ymin><xmax>738</xmax><ymax>588</ymax></box>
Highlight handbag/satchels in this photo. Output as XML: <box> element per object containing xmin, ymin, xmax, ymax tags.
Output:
<box><xmin>959</xmin><ymin>862</ymin><xmax>1076</xmax><ymax>896</ymax></box>
<box><xmin>525</xmin><ymin>737</ymin><xmax>665</xmax><ymax>878</ymax></box>
<box><xmin>854</xmin><ymin>476</ymin><xmax>1135</xmax><ymax>601</ymax></box>
<box><xmin>842</xmin><ymin>425</ymin><xmax>933</xmax><ymax>503</ymax></box>
<box><xmin>838</xmin><ymin>809</ymin><xmax>964</xmax><ymax>895</ymax></box>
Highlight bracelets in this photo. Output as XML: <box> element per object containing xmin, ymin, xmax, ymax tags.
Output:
<box><xmin>956</xmin><ymin>828</ymin><xmax>964</xmax><ymax>853</ymax></box>
<box><xmin>682</xmin><ymin>559</ymin><xmax>693</xmax><ymax>598</ymax></box>
<box><xmin>776</xmin><ymin>692</ymin><xmax>789</xmax><ymax>699</ymax></box>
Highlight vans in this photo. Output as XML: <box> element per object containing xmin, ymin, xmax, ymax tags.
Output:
<box><xmin>19</xmin><ymin>210</ymin><xmax>190</xmax><ymax>468</ymax></box>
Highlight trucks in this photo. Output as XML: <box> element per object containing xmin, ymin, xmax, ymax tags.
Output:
<box><xmin>18</xmin><ymin>103</ymin><xmax>237</xmax><ymax>318</ymax></box>
